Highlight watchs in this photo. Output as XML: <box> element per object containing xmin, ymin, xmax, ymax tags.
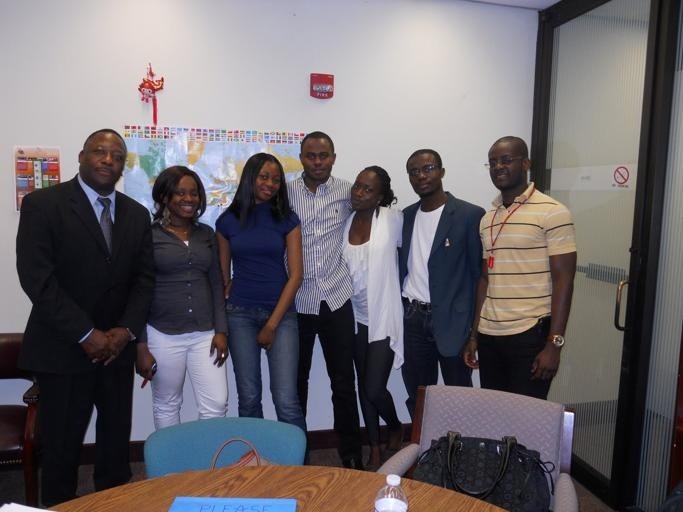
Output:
<box><xmin>545</xmin><ymin>334</ymin><xmax>565</xmax><ymax>349</ymax></box>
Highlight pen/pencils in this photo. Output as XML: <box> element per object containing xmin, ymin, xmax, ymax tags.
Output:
<box><xmin>141</xmin><ymin>364</ymin><xmax>157</xmax><ymax>388</ymax></box>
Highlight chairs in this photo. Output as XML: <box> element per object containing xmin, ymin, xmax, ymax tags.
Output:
<box><xmin>376</xmin><ymin>385</ymin><xmax>579</xmax><ymax>512</ymax></box>
<box><xmin>143</xmin><ymin>417</ymin><xmax>308</xmax><ymax>479</ymax></box>
<box><xmin>0</xmin><ymin>333</ymin><xmax>41</xmax><ymax>507</ymax></box>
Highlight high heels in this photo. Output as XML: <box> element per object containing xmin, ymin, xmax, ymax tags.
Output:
<box><xmin>364</xmin><ymin>443</ymin><xmax>384</xmax><ymax>471</ymax></box>
<box><xmin>382</xmin><ymin>425</ymin><xmax>405</xmax><ymax>462</ymax></box>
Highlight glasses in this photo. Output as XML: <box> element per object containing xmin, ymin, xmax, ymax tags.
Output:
<box><xmin>484</xmin><ymin>157</ymin><xmax>521</xmax><ymax>170</ymax></box>
<box><xmin>409</xmin><ymin>165</ymin><xmax>441</xmax><ymax>175</ymax></box>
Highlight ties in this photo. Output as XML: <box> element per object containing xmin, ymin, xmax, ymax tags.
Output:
<box><xmin>97</xmin><ymin>197</ymin><xmax>113</xmax><ymax>258</ymax></box>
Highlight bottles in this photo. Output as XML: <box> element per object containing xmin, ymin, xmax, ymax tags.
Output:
<box><xmin>374</xmin><ymin>474</ymin><xmax>408</xmax><ymax>512</ymax></box>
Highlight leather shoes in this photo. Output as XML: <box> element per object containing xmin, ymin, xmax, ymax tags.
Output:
<box><xmin>343</xmin><ymin>458</ymin><xmax>364</xmax><ymax>470</ymax></box>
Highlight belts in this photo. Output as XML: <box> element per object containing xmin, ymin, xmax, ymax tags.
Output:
<box><xmin>401</xmin><ymin>297</ymin><xmax>434</xmax><ymax>313</ymax></box>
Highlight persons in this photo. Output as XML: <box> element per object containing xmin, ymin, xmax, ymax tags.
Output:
<box><xmin>136</xmin><ymin>166</ymin><xmax>228</xmax><ymax>431</ymax></box>
<box><xmin>463</xmin><ymin>136</ymin><xmax>577</xmax><ymax>400</ymax></box>
<box><xmin>341</xmin><ymin>166</ymin><xmax>404</xmax><ymax>472</ymax></box>
<box><xmin>215</xmin><ymin>152</ymin><xmax>307</xmax><ymax>431</ymax></box>
<box><xmin>16</xmin><ymin>129</ymin><xmax>155</xmax><ymax>508</ymax></box>
<box><xmin>397</xmin><ymin>148</ymin><xmax>486</xmax><ymax>423</ymax></box>
<box><xmin>225</xmin><ymin>131</ymin><xmax>364</xmax><ymax>470</ymax></box>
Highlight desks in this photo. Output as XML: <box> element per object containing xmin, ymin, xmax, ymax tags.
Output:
<box><xmin>49</xmin><ymin>466</ymin><xmax>508</xmax><ymax>512</ymax></box>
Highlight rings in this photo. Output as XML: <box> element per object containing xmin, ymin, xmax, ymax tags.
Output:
<box><xmin>222</xmin><ymin>353</ymin><xmax>226</xmax><ymax>359</ymax></box>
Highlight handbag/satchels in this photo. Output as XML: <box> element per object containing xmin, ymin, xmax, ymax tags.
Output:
<box><xmin>403</xmin><ymin>430</ymin><xmax>552</xmax><ymax>507</ymax></box>
<box><xmin>209</xmin><ymin>437</ymin><xmax>269</xmax><ymax>469</ymax></box>
